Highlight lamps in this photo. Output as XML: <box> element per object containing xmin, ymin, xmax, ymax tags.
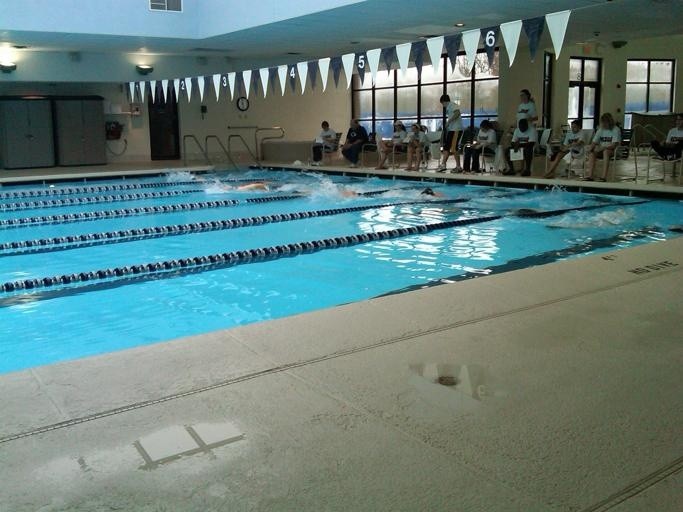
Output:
<box><xmin>0</xmin><ymin>63</ymin><xmax>17</xmax><ymax>73</ymax></box>
<box><xmin>136</xmin><ymin>65</ymin><xmax>153</xmax><ymax>75</ymax></box>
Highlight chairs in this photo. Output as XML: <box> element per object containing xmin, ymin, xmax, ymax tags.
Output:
<box><xmin>309</xmin><ymin>122</ymin><xmax>682</xmax><ymax>184</ymax></box>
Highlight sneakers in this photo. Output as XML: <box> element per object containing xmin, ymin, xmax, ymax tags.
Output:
<box><xmin>582</xmin><ymin>177</ymin><xmax>608</xmax><ymax>182</ymax></box>
<box><xmin>312</xmin><ymin>161</ymin><xmax>320</xmax><ymax>166</ymax></box>
<box><xmin>502</xmin><ymin>170</ymin><xmax>531</xmax><ymax>176</ymax></box>
<box><xmin>667</xmin><ymin>153</ymin><xmax>676</xmax><ymax>160</ymax></box>
<box><xmin>349</xmin><ymin>160</ymin><xmax>360</xmax><ymax>168</ymax></box>
<box><xmin>436</xmin><ymin>166</ymin><xmax>462</xmax><ymax>173</ymax></box>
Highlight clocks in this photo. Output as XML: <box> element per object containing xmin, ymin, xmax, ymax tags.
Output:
<box><xmin>238</xmin><ymin>97</ymin><xmax>249</xmax><ymax>110</ymax></box>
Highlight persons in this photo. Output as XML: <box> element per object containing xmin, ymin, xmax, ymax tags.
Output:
<box><xmin>580</xmin><ymin>112</ymin><xmax>621</xmax><ymax>182</ymax></box>
<box><xmin>230</xmin><ymin>179</ymin><xmax>274</xmax><ymax>192</ymax></box>
<box><xmin>374</xmin><ymin>119</ymin><xmax>406</xmax><ymax>170</ymax></box>
<box><xmin>514</xmin><ymin>88</ymin><xmax>539</xmax><ymax>133</ymax></box>
<box><xmin>434</xmin><ymin>93</ymin><xmax>465</xmax><ymax>175</ymax></box>
<box><xmin>542</xmin><ymin>118</ymin><xmax>586</xmax><ymax>180</ymax></box>
<box><xmin>421</xmin><ymin>125</ymin><xmax>429</xmax><ymax>152</ymax></box>
<box><xmin>341</xmin><ymin>119</ymin><xmax>368</xmax><ymax>168</ymax></box>
<box><xmin>310</xmin><ymin>120</ymin><xmax>336</xmax><ymax>167</ymax></box>
<box><xmin>503</xmin><ymin>118</ymin><xmax>539</xmax><ymax>179</ymax></box>
<box><xmin>649</xmin><ymin>112</ymin><xmax>682</xmax><ymax>160</ymax></box>
<box><xmin>421</xmin><ymin>187</ymin><xmax>442</xmax><ymax>198</ymax></box>
<box><xmin>402</xmin><ymin>123</ymin><xmax>427</xmax><ymax>172</ymax></box>
<box><xmin>460</xmin><ymin>118</ymin><xmax>496</xmax><ymax>174</ymax></box>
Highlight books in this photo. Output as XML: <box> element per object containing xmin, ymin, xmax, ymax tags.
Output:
<box><xmin>313</xmin><ymin>136</ymin><xmax>324</xmax><ymax>145</ymax></box>
<box><xmin>509</xmin><ymin>146</ymin><xmax>524</xmax><ymax>163</ymax></box>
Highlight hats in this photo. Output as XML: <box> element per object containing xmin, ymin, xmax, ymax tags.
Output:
<box><xmin>394</xmin><ymin>121</ymin><xmax>402</xmax><ymax>126</ymax></box>
